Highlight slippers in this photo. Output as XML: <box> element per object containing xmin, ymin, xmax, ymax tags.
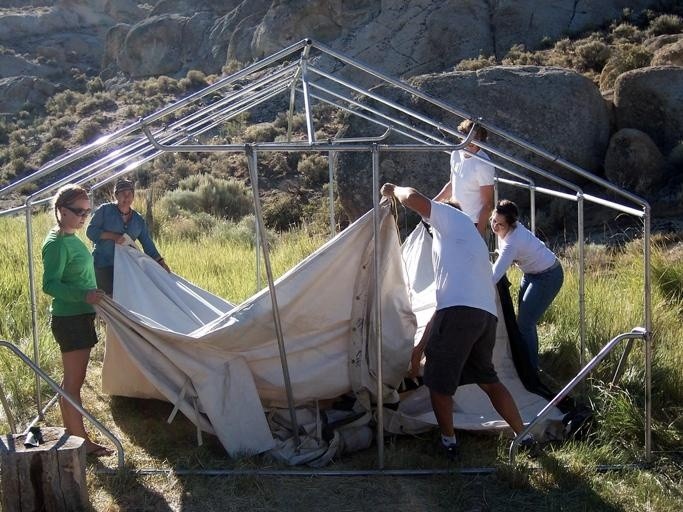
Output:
<box><xmin>85</xmin><ymin>444</ymin><xmax>113</xmax><ymax>456</ymax></box>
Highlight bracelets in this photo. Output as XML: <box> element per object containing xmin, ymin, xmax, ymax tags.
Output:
<box><xmin>157</xmin><ymin>258</ymin><xmax>163</xmax><ymax>263</ymax></box>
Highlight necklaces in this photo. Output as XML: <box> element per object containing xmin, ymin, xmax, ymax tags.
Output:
<box><xmin>117</xmin><ymin>206</ymin><xmax>133</xmax><ymax>216</ymax></box>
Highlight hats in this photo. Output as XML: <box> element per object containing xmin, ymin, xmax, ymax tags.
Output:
<box><xmin>116</xmin><ymin>179</ymin><xmax>134</xmax><ymax>194</ymax></box>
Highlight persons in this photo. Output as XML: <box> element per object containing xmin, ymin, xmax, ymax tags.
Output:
<box><xmin>41</xmin><ymin>184</ymin><xmax>112</xmax><ymax>456</ymax></box>
<box><xmin>381</xmin><ymin>182</ymin><xmax>535</xmax><ymax>455</ymax></box>
<box><xmin>431</xmin><ymin>120</ymin><xmax>496</xmax><ymax>243</ymax></box>
<box><xmin>86</xmin><ymin>177</ymin><xmax>170</xmax><ymax>294</ymax></box>
<box><xmin>492</xmin><ymin>199</ymin><xmax>564</xmax><ymax>366</ymax></box>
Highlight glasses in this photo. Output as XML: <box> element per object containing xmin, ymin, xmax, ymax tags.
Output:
<box><xmin>63</xmin><ymin>206</ymin><xmax>91</xmax><ymax>215</ymax></box>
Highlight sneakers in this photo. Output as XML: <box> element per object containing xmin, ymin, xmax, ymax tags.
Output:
<box><xmin>518</xmin><ymin>437</ymin><xmax>538</xmax><ymax>457</ymax></box>
<box><xmin>432</xmin><ymin>438</ymin><xmax>457</xmax><ymax>458</ymax></box>
